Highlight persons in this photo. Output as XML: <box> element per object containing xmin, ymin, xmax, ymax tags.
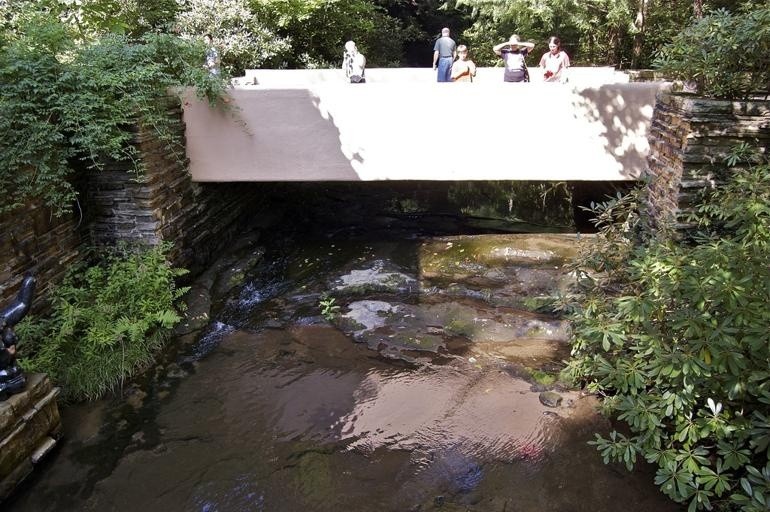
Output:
<box><xmin>431</xmin><ymin>27</ymin><xmax>458</xmax><ymax>82</ymax></box>
<box><xmin>202</xmin><ymin>33</ymin><xmax>223</xmax><ymax>87</ymax></box>
<box><xmin>539</xmin><ymin>36</ymin><xmax>571</xmax><ymax>83</ymax></box>
<box><xmin>451</xmin><ymin>44</ymin><xmax>478</xmax><ymax>82</ymax></box>
<box><xmin>0</xmin><ymin>316</ymin><xmax>30</xmax><ymax>403</ymax></box>
<box><xmin>493</xmin><ymin>34</ymin><xmax>535</xmax><ymax>82</ymax></box>
<box><xmin>341</xmin><ymin>40</ymin><xmax>367</xmax><ymax>84</ymax></box>
